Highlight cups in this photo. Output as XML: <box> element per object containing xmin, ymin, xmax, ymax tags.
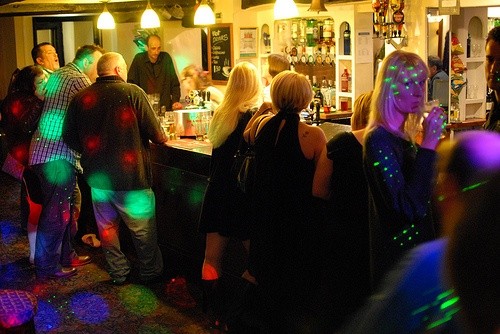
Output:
<box><xmin>193</xmin><ymin>116</ymin><xmax>211</xmax><ymax>140</ymax></box>
<box><xmin>158</xmin><ymin>116</ymin><xmax>180</xmax><ymax>141</ymax></box>
<box><xmin>341</xmin><ymin>102</ymin><xmax>348</xmax><ymax>111</ymax></box>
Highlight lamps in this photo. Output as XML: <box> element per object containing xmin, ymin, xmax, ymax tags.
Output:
<box><xmin>97</xmin><ymin>0</ymin><xmax>115</xmax><ymax>29</ymax></box>
<box><xmin>141</xmin><ymin>0</ymin><xmax>160</xmax><ymax>28</ymax></box>
<box><xmin>273</xmin><ymin>0</ymin><xmax>299</xmax><ymax>20</ymax></box>
<box><xmin>194</xmin><ymin>0</ymin><xmax>215</xmax><ymax>25</ymax></box>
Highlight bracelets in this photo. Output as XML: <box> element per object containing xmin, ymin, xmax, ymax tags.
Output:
<box><xmin>257</xmin><ymin>109</ymin><xmax>262</xmax><ymax>116</ymax></box>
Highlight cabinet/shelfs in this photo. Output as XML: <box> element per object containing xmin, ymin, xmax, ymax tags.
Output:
<box><xmin>334</xmin><ymin>4</ymin><xmax>373</xmax><ymax>113</ymax></box>
<box><xmin>451</xmin><ymin>8</ymin><xmax>487</xmax><ymax>121</ymax></box>
<box><xmin>257</xmin><ymin>10</ymin><xmax>292</xmax><ymax>100</ymax></box>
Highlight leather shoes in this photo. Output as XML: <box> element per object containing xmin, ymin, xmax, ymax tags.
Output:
<box><xmin>61</xmin><ymin>255</ymin><xmax>93</xmax><ymax>267</ymax></box>
<box><xmin>35</xmin><ymin>268</ymin><xmax>79</xmax><ymax>279</ymax></box>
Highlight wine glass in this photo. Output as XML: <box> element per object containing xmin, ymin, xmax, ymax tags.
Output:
<box><xmin>472</xmin><ymin>45</ymin><xmax>481</xmax><ymax>58</ymax></box>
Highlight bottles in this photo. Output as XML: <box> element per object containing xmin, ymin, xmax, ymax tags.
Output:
<box><xmin>278</xmin><ymin>18</ymin><xmax>335</xmax><ymax>55</ymax></box>
<box><xmin>305</xmin><ymin>74</ymin><xmax>336</xmax><ymax>110</ymax></box>
<box><xmin>342</xmin><ymin>68</ymin><xmax>349</xmax><ymax>91</ymax></box>
<box><xmin>450</xmin><ymin>102</ymin><xmax>460</xmax><ymax>120</ymax></box>
<box><xmin>344</xmin><ymin>23</ymin><xmax>351</xmax><ymax>55</ymax></box>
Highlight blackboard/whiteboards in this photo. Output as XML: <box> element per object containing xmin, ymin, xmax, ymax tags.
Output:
<box><xmin>207</xmin><ymin>23</ymin><xmax>235</xmax><ymax>86</ymax></box>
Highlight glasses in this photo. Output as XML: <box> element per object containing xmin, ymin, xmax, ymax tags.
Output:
<box><xmin>406</xmin><ymin>79</ymin><xmax>425</xmax><ymax>93</ymax></box>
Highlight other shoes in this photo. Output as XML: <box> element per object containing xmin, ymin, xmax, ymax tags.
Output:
<box><xmin>137</xmin><ymin>269</ymin><xmax>162</xmax><ymax>285</ymax></box>
<box><xmin>201</xmin><ymin>258</ymin><xmax>232</xmax><ymax>281</ymax></box>
<box><xmin>110</xmin><ymin>275</ymin><xmax>130</xmax><ymax>286</ymax></box>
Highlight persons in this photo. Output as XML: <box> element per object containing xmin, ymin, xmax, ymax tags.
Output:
<box><xmin>127</xmin><ymin>34</ymin><xmax>182</xmax><ymax>112</ymax></box>
<box><xmin>426</xmin><ymin>55</ymin><xmax>449</xmax><ymax>102</ymax></box>
<box><xmin>312</xmin><ymin>89</ymin><xmax>374</xmax><ymax>248</ymax></box>
<box><xmin>28</xmin><ymin>45</ymin><xmax>105</xmax><ymax>279</ymax></box>
<box><xmin>62</xmin><ymin>54</ymin><xmax>168</xmax><ymax>285</ymax></box>
<box><xmin>356</xmin><ymin>130</ymin><xmax>500</xmax><ymax>334</ymax></box>
<box><xmin>243</xmin><ymin>70</ymin><xmax>329</xmax><ymax>281</ymax></box>
<box><xmin>362</xmin><ymin>50</ymin><xmax>446</xmax><ymax>305</ymax></box>
<box><xmin>201</xmin><ymin>67</ymin><xmax>264</xmax><ymax>313</ymax></box>
<box><xmin>479</xmin><ymin>26</ymin><xmax>500</xmax><ymax>133</ymax></box>
<box><xmin>1</xmin><ymin>42</ymin><xmax>82</xmax><ymax>270</ymax></box>
